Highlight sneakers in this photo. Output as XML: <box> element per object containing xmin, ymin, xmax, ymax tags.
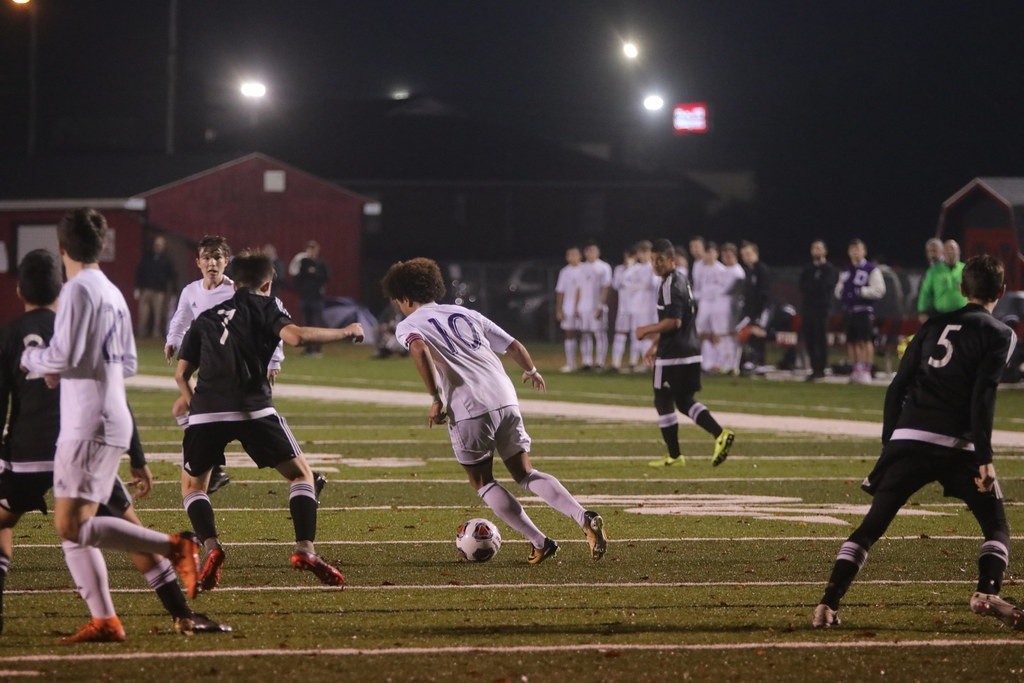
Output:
<box><xmin>313</xmin><ymin>473</ymin><xmax>327</xmax><ymax>498</ymax></box>
<box><xmin>197</xmin><ymin>543</ymin><xmax>226</xmax><ymax>591</ymax></box>
<box><xmin>648</xmin><ymin>454</ymin><xmax>685</xmax><ymax>468</ymax></box>
<box><xmin>290</xmin><ymin>547</ymin><xmax>344</xmax><ymax>586</ymax></box>
<box><xmin>711</xmin><ymin>428</ymin><xmax>735</xmax><ymax>467</ymax></box>
<box><xmin>528</xmin><ymin>537</ymin><xmax>558</xmax><ymax>564</ymax></box>
<box><xmin>581</xmin><ymin>510</ymin><xmax>608</xmax><ymax>561</ymax></box>
<box><xmin>168</xmin><ymin>530</ymin><xmax>200</xmax><ymax>599</ymax></box>
<box><xmin>58</xmin><ymin>616</ymin><xmax>125</xmax><ymax>646</ymax></box>
<box><xmin>970</xmin><ymin>592</ymin><xmax>1024</xmax><ymax>630</ymax></box>
<box><xmin>206</xmin><ymin>468</ymin><xmax>229</xmax><ymax>494</ymax></box>
<box><xmin>175</xmin><ymin>612</ymin><xmax>232</xmax><ymax>634</ymax></box>
<box><xmin>812</xmin><ymin>604</ymin><xmax>841</xmax><ymax>628</ymax></box>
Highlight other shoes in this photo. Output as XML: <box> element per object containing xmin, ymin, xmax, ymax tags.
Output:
<box><xmin>850</xmin><ymin>372</ymin><xmax>870</xmax><ymax>383</ymax></box>
<box><xmin>805</xmin><ymin>372</ymin><xmax>828</xmax><ymax>381</ymax></box>
<box><xmin>560</xmin><ymin>359</ymin><xmax>770</xmax><ymax>377</ymax></box>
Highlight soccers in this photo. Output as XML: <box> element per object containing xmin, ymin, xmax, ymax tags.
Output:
<box><xmin>455</xmin><ymin>518</ymin><xmax>503</xmax><ymax>564</ymax></box>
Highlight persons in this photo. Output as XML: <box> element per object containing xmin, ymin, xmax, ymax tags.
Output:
<box><xmin>19</xmin><ymin>209</ymin><xmax>205</xmax><ymax>647</ymax></box>
<box><xmin>172</xmin><ymin>249</ymin><xmax>367</xmax><ymax>589</ymax></box>
<box><xmin>926</xmin><ymin>238</ymin><xmax>945</xmax><ymax>266</ymax></box>
<box><xmin>0</xmin><ymin>249</ymin><xmax>233</xmax><ymax>635</ymax></box>
<box><xmin>289</xmin><ymin>242</ymin><xmax>327</xmax><ymax>355</ymax></box>
<box><xmin>636</xmin><ymin>239</ymin><xmax>735</xmax><ymax>469</ymax></box>
<box><xmin>800</xmin><ymin>242</ymin><xmax>838</xmax><ymax>379</ymax></box>
<box><xmin>918</xmin><ymin>240</ymin><xmax>968</xmax><ymax>323</ymax></box>
<box><xmin>132</xmin><ymin>233</ymin><xmax>175</xmax><ymax>339</ymax></box>
<box><xmin>165</xmin><ymin>236</ymin><xmax>327</xmax><ymax>510</ymax></box>
<box><xmin>555</xmin><ymin>240</ymin><xmax>784</xmax><ymax>375</ymax></box>
<box><xmin>834</xmin><ymin>240</ymin><xmax>887</xmax><ymax>385</ymax></box>
<box><xmin>383</xmin><ymin>257</ymin><xmax>607</xmax><ymax>565</ymax></box>
<box><xmin>813</xmin><ymin>255</ymin><xmax>1024</xmax><ymax>629</ymax></box>
